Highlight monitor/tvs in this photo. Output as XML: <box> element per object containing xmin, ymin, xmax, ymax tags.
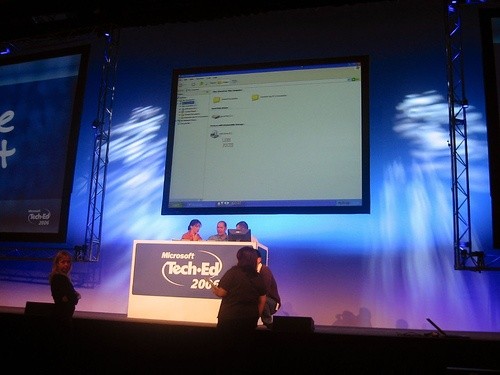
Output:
<box><xmin>228</xmin><ymin>229</ymin><xmax>251</xmax><ymax>242</ymax></box>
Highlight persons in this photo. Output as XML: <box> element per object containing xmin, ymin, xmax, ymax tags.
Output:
<box><xmin>206</xmin><ymin>247</ymin><xmax>267</xmax><ymax>375</ymax></box>
<box><xmin>208</xmin><ymin>221</ymin><xmax>228</xmax><ymax>241</ymax></box>
<box><xmin>256</xmin><ymin>250</ymin><xmax>281</xmax><ymax>329</ymax></box>
<box><xmin>236</xmin><ymin>221</ymin><xmax>257</xmax><ymax>242</ymax></box>
<box><xmin>181</xmin><ymin>219</ymin><xmax>204</xmax><ymax>241</ymax></box>
<box><xmin>50</xmin><ymin>251</ymin><xmax>81</xmax><ymax>375</ymax></box>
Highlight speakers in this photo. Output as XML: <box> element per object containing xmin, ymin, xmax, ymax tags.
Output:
<box><xmin>25</xmin><ymin>301</ymin><xmax>55</xmax><ymax>315</ymax></box>
<box><xmin>273</xmin><ymin>315</ymin><xmax>314</xmax><ymax>332</ymax></box>
